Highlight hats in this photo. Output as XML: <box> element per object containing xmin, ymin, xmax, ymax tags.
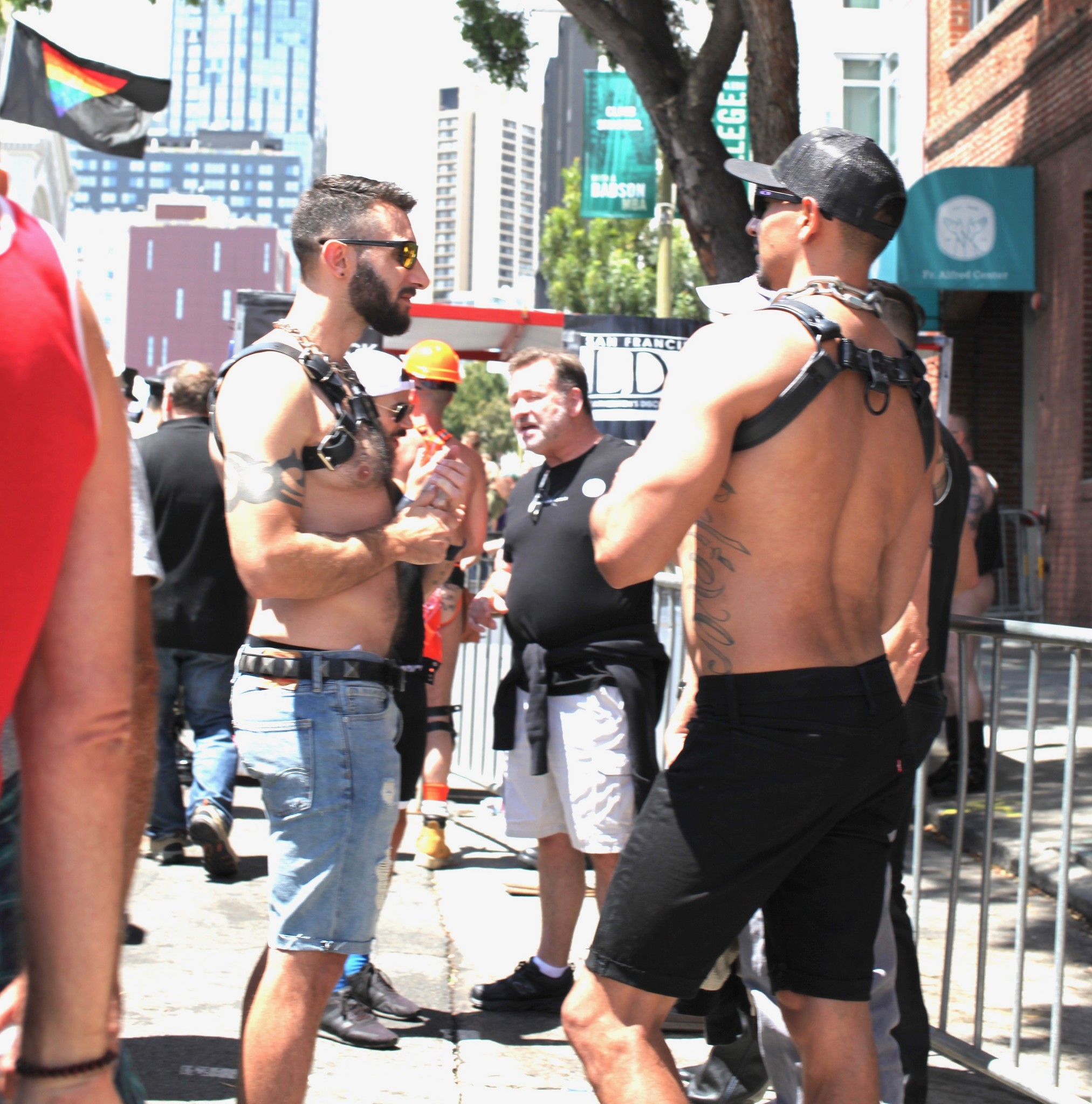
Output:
<box><xmin>724</xmin><ymin>126</ymin><xmax>907</xmax><ymax>241</ymax></box>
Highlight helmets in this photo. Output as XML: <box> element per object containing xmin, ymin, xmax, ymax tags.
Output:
<box><xmin>402</xmin><ymin>339</ymin><xmax>464</xmax><ymax>385</ymax></box>
<box><xmin>344</xmin><ymin>348</ymin><xmax>416</xmax><ymax>397</ymax></box>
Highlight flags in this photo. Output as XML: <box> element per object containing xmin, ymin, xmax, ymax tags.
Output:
<box><xmin>0</xmin><ymin>17</ymin><xmax>172</xmax><ymax>160</ymax></box>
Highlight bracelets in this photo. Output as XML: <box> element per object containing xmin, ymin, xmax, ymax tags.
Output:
<box><xmin>14</xmin><ymin>1052</ymin><xmax>119</xmax><ymax>1079</ymax></box>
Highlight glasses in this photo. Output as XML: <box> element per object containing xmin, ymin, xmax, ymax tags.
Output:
<box><xmin>372</xmin><ymin>402</ymin><xmax>416</xmax><ymax>422</ymax></box>
<box><xmin>751</xmin><ymin>186</ymin><xmax>834</xmax><ymax>222</ymax></box>
<box><xmin>319</xmin><ymin>238</ymin><xmax>418</xmax><ymax>270</ymax></box>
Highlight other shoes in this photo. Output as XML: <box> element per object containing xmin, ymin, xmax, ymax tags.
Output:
<box><xmin>412</xmin><ymin>816</ymin><xmax>453</xmax><ymax>870</ymax></box>
<box><xmin>656</xmin><ymin>993</ymin><xmax>708</xmax><ymax>1033</ymax></box>
<box><xmin>519</xmin><ymin>844</ymin><xmax>595</xmax><ymax>870</ymax></box>
<box><xmin>504</xmin><ymin>869</ymin><xmax>597</xmax><ymax>899</ymax></box>
<box><xmin>927</xmin><ymin>749</ymin><xmax>991</xmax><ymax>796</ymax></box>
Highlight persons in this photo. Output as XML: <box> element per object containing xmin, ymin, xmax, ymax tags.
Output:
<box><xmin>0</xmin><ymin>126</ymin><xmax>1005</xmax><ymax>1104</ymax></box>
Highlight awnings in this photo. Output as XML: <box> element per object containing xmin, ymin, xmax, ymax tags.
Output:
<box><xmin>868</xmin><ymin>164</ymin><xmax>1036</xmax><ymax>331</ymax></box>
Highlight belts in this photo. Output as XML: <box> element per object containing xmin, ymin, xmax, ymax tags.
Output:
<box><xmin>239</xmin><ymin>652</ymin><xmax>410</xmax><ymax>695</ymax></box>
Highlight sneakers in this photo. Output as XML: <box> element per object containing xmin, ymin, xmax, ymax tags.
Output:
<box><xmin>316</xmin><ymin>963</ymin><xmax>423</xmax><ymax>1049</ymax></box>
<box><xmin>470</xmin><ymin>956</ymin><xmax>574</xmax><ymax>1014</ymax></box>
<box><xmin>138</xmin><ymin>831</ymin><xmax>186</xmax><ymax>866</ymax></box>
<box><xmin>188</xmin><ymin>803</ymin><xmax>242</xmax><ymax>883</ymax></box>
